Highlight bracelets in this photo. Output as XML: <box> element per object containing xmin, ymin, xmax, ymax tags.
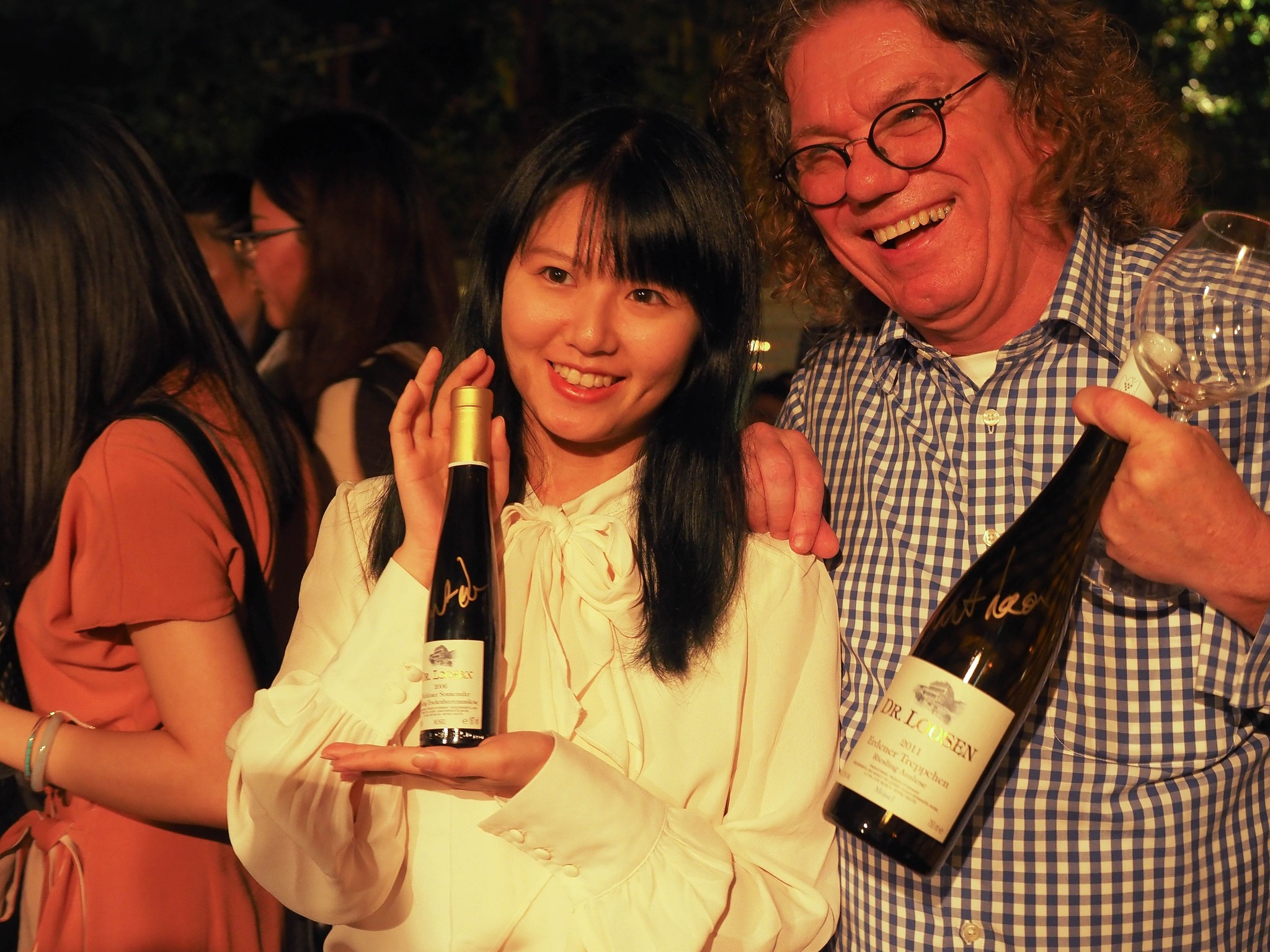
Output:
<box><xmin>31</xmin><ymin>712</ymin><xmax>65</xmax><ymax>792</ymax></box>
<box><xmin>24</xmin><ymin>708</ymin><xmax>96</xmax><ymax>783</ymax></box>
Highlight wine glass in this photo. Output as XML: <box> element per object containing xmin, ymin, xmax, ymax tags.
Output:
<box><xmin>1081</xmin><ymin>210</ymin><xmax>1270</xmax><ymax>612</ymax></box>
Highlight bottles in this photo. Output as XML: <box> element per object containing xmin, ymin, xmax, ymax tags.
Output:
<box><xmin>824</xmin><ymin>330</ymin><xmax>1188</xmax><ymax>878</ymax></box>
<box><xmin>418</xmin><ymin>386</ymin><xmax>500</xmax><ymax>747</ymax></box>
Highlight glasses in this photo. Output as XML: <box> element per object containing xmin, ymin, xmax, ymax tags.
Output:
<box><xmin>230</xmin><ymin>225</ymin><xmax>303</xmax><ymax>269</ymax></box>
<box><xmin>780</xmin><ymin>69</ymin><xmax>992</xmax><ymax>211</ymax></box>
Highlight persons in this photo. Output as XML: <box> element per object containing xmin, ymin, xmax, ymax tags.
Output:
<box><xmin>249</xmin><ymin>103</ymin><xmax>479</xmax><ymax>528</ymax></box>
<box><xmin>0</xmin><ymin>87</ymin><xmax>339</xmax><ymax>952</ymax></box>
<box><xmin>222</xmin><ymin>105</ymin><xmax>849</xmax><ymax>952</ymax></box>
<box><xmin>678</xmin><ymin>0</ymin><xmax>1270</xmax><ymax>952</ymax></box>
<box><xmin>148</xmin><ymin>174</ymin><xmax>324</xmax><ymax>485</ymax></box>
